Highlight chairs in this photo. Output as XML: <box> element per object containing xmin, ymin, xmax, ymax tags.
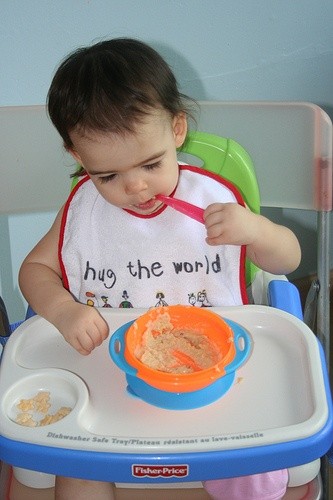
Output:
<box><xmin>0</xmin><ymin>101</ymin><xmax>333</xmax><ymax>500</ymax></box>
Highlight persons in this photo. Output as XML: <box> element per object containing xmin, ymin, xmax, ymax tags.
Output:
<box><xmin>19</xmin><ymin>37</ymin><xmax>302</xmax><ymax>500</ymax></box>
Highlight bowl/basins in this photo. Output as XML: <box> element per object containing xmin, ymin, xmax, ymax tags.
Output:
<box><xmin>109</xmin><ymin>306</ymin><xmax>250</xmax><ymax>411</ymax></box>
